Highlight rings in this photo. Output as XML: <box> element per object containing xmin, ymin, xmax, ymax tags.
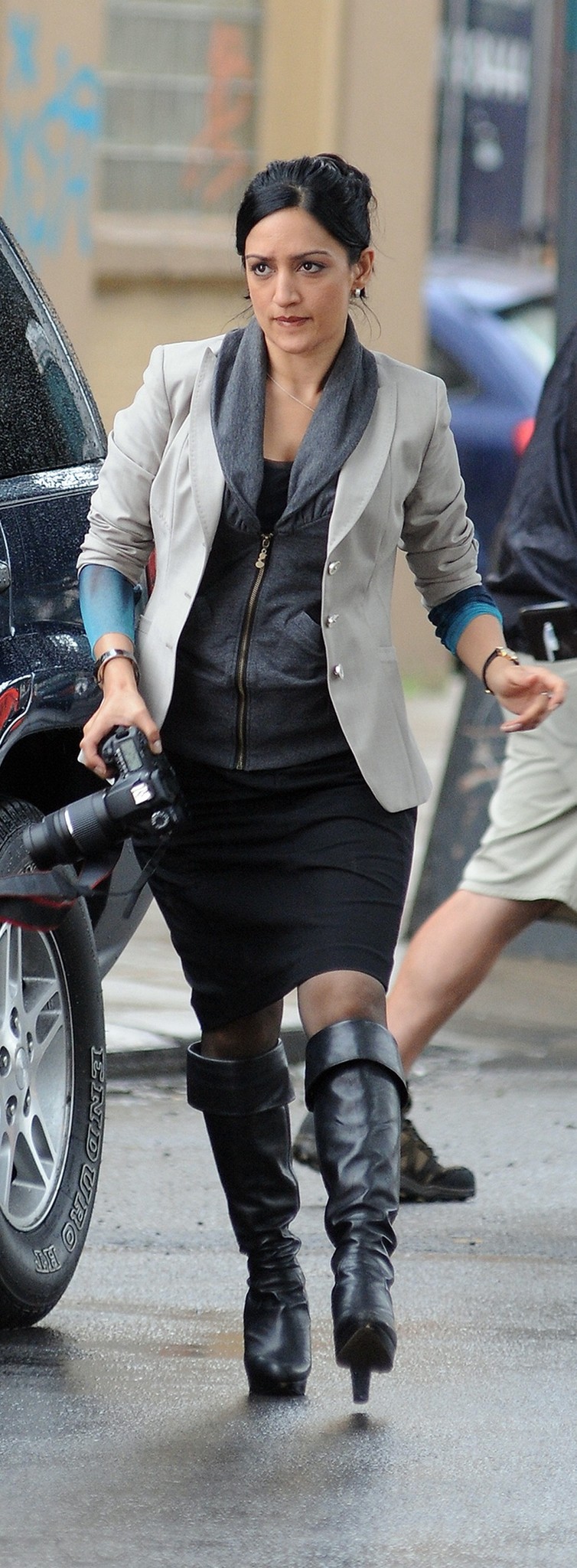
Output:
<box><xmin>542</xmin><ymin>692</ymin><xmax>552</xmax><ymax>700</ymax></box>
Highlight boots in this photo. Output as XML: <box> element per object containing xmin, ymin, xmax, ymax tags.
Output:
<box><xmin>303</xmin><ymin>1019</ymin><xmax>408</xmax><ymax>1405</ymax></box>
<box><xmin>186</xmin><ymin>1038</ymin><xmax>312</xmax><ymax>1396</ymax></box>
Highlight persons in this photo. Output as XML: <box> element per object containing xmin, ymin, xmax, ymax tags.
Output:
<box><xmin>76</xmin><ymin>155</ymin><xmax>568</xmax><ymax>1407</ymax></box>
<box><xmin>292</xmin><ymin>328</ymin><xmax>577</xmax><ymax>1203</ymax></box>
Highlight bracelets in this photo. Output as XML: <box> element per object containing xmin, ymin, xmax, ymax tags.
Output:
<box><xmin>93</xmin><ymin>650</ymin><xmax>139</xmax><ymax>688</ymax></box>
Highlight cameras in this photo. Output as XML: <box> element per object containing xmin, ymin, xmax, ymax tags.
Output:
<box><xmin>24</xmin><ymin>724</ymin><xmax>186</xmax><ymax>870</ymax></box>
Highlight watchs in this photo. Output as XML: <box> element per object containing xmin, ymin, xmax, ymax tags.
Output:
<box><xmin>483</xmin><ymin>647</ymin><xmax>519</xmax><ymax>696</ymax></box>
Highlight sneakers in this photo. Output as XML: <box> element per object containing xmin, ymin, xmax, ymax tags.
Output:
<box><xmin>293</xmin><ymin>1107</ymin><xmax>477</xmax><ymax>1202</ymax></box>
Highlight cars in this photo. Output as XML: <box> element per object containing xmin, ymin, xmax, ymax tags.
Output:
<box><xmin>0</xmin><ymin>204</ymin><xmax>166</xmax><ymax>1326</ymax></box>
<box><xmin>422</xmin><ymin>267</ymin><xmax>562</xmax><ymax>567</ymax></box>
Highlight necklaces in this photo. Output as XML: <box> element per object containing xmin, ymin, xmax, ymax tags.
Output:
<box><xmin>266</xmin><ymin>373</ymin><xmax>316</xmax><ymax>414</ymax></box>
